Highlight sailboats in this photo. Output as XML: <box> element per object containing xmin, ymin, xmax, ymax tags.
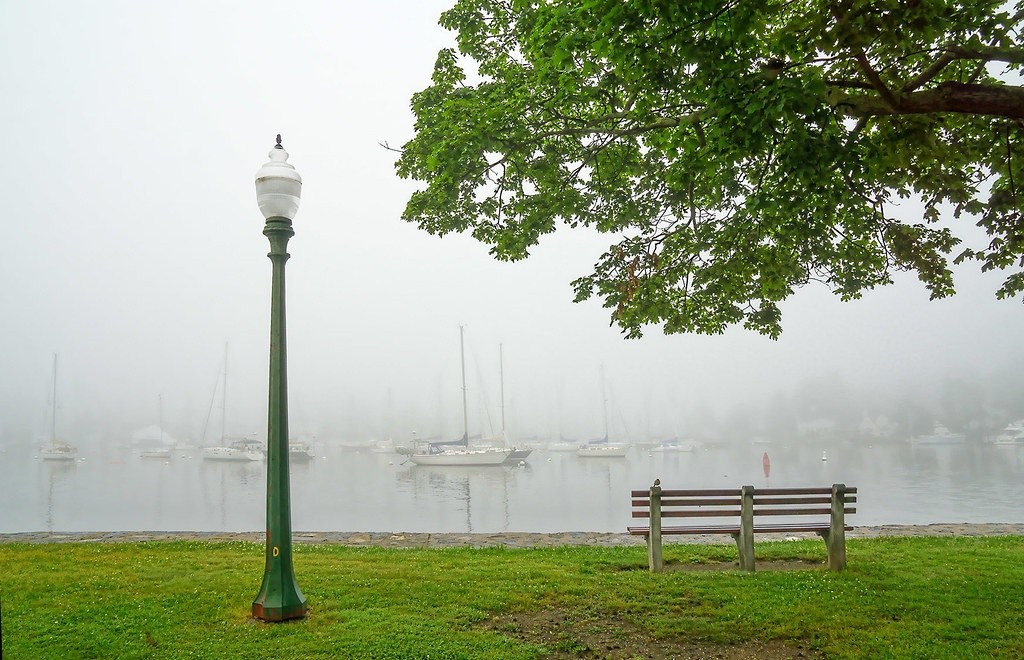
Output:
<box><xmin>375</xmin><ymin>321</ymin><xmax>693</xmax><ymax>469</ymax></box>
<box><xmin>38</xmin><ymin>350</ymin><xmax>78</xmax><ymax>462</ymax></box>
<box><xmin>135</xmin><ymin>394</ymin><xmax>172</xmax><ymax>459</ymax></box>
<box><xmin>196</xmin><ymin>339</ymin><xmax>320</xmax><ymax>464</ymax></box>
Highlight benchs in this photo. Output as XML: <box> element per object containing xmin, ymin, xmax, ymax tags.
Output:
<box><xmin>626</xmin><ymin>483</ymin><xmax>859</xmax><ymax>574</ymax></box>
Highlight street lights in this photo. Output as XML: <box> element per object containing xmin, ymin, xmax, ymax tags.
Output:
<box><xmin>250</xmin><ymin>133</ymin><xmax>308</xmax><ymax>623</ymax></box>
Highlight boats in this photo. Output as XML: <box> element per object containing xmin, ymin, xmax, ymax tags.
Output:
<box><xmin>910</xmin><ymin>431</ymin><xmax>972</xmax><ymax>447</ymax></box>
<box><xmin>991</xmin><ymin>418</ymin><xmax>1024</xmax><ymax>447</ymax></box>
